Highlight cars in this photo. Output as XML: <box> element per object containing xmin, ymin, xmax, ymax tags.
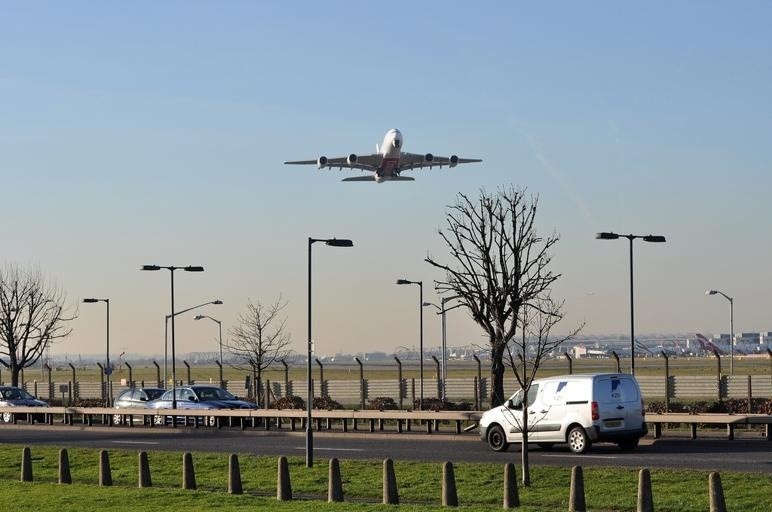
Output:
<box><xmin>110</xmin><ymin>383</ymin><xmax>168</xmax><ymax>426</ymax></box>
<box><xmin>143</xmin><ymin>383</ymin><xmax>260</xmax><ymax>428</ymax></box>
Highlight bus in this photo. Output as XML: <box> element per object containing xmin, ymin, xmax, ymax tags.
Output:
<box><xmin>0</xmin><ymin>385</ymin><xmax>51</xmax><ymax>424</ymax></box>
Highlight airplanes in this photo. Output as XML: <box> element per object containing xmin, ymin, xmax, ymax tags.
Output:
<box><xmin>283</xmin><ymin>127</ymin><xmax>483</xmax><ymax>183</ymax></box>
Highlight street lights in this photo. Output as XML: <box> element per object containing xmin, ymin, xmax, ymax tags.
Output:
<box><xmin>421</xmin><ymin>297</ymin><xmax>449</xmax><ymax>402</ymax></box>
<box><xmin>705</xmin><ymin>288</ymin><xmax>734</xmax><ymax>376</ymax></box>
<box><xmin>191</xmin><ymin>313</ymin><xmax>222</xmax><ymax>388</ymax></box>
<box><xmin>164</xmin><ymin>299</ymin><xmax>223</xmax><ymax>389</ymax></box>
<box><xmin>81</xmin><ymin>298</ymin><xmax>110</xmax><ymax>410</ymax></box>
<box><xmin>593</xmin><ymin>232</ymin><xmax>666</xmax><ymax>380</ymax></box>
<box><xmin>306</xmin><ymin>236</ymin><xmax>355</xmax><ymax>468</ymax></box>
<box><xmin>19</xmin><ymin>324</ymin><xmax>44</xmax><ymax>383</ymax></box>
<box><xmin>394</xmin><ymin>279</ymin><xmax>423</xmax><ymax>410</ymax></box>
<box><xmin>139</xmin><ymin>264</ymin><xmax>205</xmax><ymax>429</ymax></box>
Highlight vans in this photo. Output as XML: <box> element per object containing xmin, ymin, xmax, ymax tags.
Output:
<box><xmin>479</xmin><ymin>373</ymin><xmax>648</xmax><ymax>455</ymax></box>
